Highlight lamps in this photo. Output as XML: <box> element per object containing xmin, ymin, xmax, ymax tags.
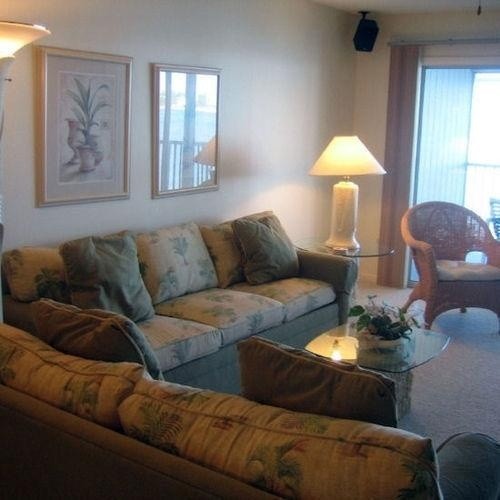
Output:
<box><xmin>191</xmin><ymin>134</ymin><xmax>219</xmax><ymax>183</ymax></box>
<box><xmin>308</xmin><ymin>132</ymin><xmax>390</xmax><ymax>254</ymax></box>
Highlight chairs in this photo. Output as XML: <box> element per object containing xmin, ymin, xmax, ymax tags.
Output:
<box><xmin>401</xmin><ymin>200</ymin><xmax>500</xmax><ymax>329</ymax></box>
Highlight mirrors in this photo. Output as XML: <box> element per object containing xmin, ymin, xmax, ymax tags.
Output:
<box><xmin>148</xmin><ymin>64</ymin><xmax>220</xmax><ymax>199</ymax></box>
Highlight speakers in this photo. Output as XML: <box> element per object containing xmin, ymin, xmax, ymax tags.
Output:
<box><xmin>352</xmin><ymin>18</ymin><xmax>380</xmax><ymax>52</ymax></box>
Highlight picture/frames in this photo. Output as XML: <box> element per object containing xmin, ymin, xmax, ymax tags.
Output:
<box><xmin>36</xmin><ymin>44</ymin><xmax>134</xmax><ymax>205</ymax></box>
